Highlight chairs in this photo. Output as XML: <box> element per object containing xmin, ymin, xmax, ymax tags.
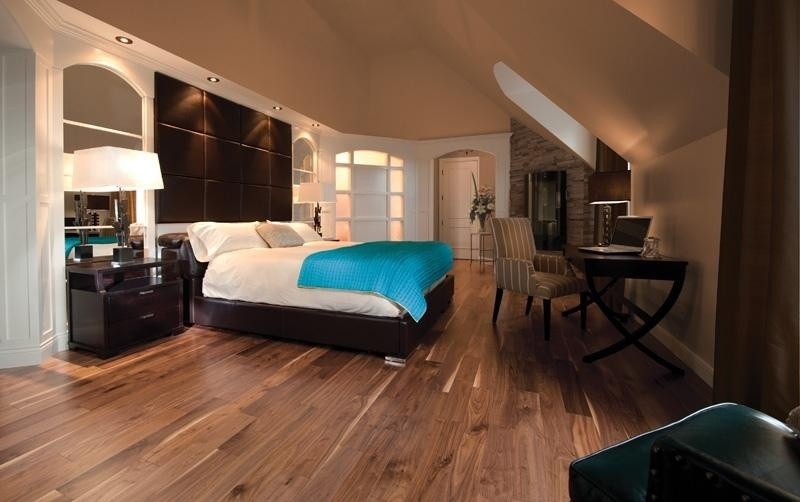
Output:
<box><xmin>569</xmin><ymin>401</ymin><xmax>799</xmax><ymax>502</ymax></box>
<box><xmin>489</xmin><ymin>218</ymin><xmax>587</xmax><ymax>340</ymax></box>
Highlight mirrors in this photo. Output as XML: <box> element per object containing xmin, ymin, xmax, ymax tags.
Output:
<box><xmin>63</xmin><ymin>63</ymin><xmax>146</xmax><ymax>266</ymax></box>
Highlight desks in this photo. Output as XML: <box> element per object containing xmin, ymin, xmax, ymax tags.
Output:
<box><xmin>471</xmin><ymin>233</ymin><xmax>495</xmax><ymax>272</ymax></box>
<box><xmin>561</xmin><ymin>245</ymin><xmax>688</xmax><ymax>376</ymax></box>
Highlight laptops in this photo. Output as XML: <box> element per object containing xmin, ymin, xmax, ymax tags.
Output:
<box><xmin>576</xmin><ymin>215</ymin><xmax>653</xmax><ymax>254</ymax></box>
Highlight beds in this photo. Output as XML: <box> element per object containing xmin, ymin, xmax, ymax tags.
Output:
<box><xmin>65</xmin><ymin>235</ymin><xmax>128</xmax><ymax>259</ymax></box>
<box><xmin>158</xmin><ymin>233</ymin><xmax>454</xmax><ymax>363</ymax></box>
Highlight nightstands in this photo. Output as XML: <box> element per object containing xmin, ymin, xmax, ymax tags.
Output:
<box><xmin>66</xmin><ymin>258</ymin><xmax>184</xmax><ymax>360</ymax></box>
<box><xmin>324</xmin><ymin>238</ymin><xmax>340</xmax><ymax>241</ymax></box>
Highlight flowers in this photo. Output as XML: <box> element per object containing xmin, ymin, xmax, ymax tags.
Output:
<box><xmin>468</xmin><ymin>172</ymin><xmax>495</xmax><ymax>229</ymax></box>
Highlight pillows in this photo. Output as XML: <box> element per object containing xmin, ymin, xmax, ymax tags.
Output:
<box><xmin>255</xmin><ymin>225</ymin><xmax>305</xmax><ymax>248</ymax></box>
<box><xmin>186</xmin><ymin>220</ymin><xmax>323</xmax><ymax>263</ymax></box>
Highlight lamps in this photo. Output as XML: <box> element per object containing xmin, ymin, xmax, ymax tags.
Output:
<box><xmin>71</xmin><ymin>145</ymin><xmax>164</xmax><ymax>265</ymax></box>
<box><xmin>298</xmin><ymin>183</ymin><xmax>336</xmax><ymax>237</ymax></box>
<box><xmin>588</xmin><ymin>170</ymin><xmax>631</xmax><ymax>246</ymax></box>
<box><xmin>293</xmin><ymin>185</ymin><xmax>301</xmax><ymax>204</ymax></box>
<box><xmin>64</xmin><ymin>153</ymin><xmax>93</xmax><ymax>262</ymax></box>
